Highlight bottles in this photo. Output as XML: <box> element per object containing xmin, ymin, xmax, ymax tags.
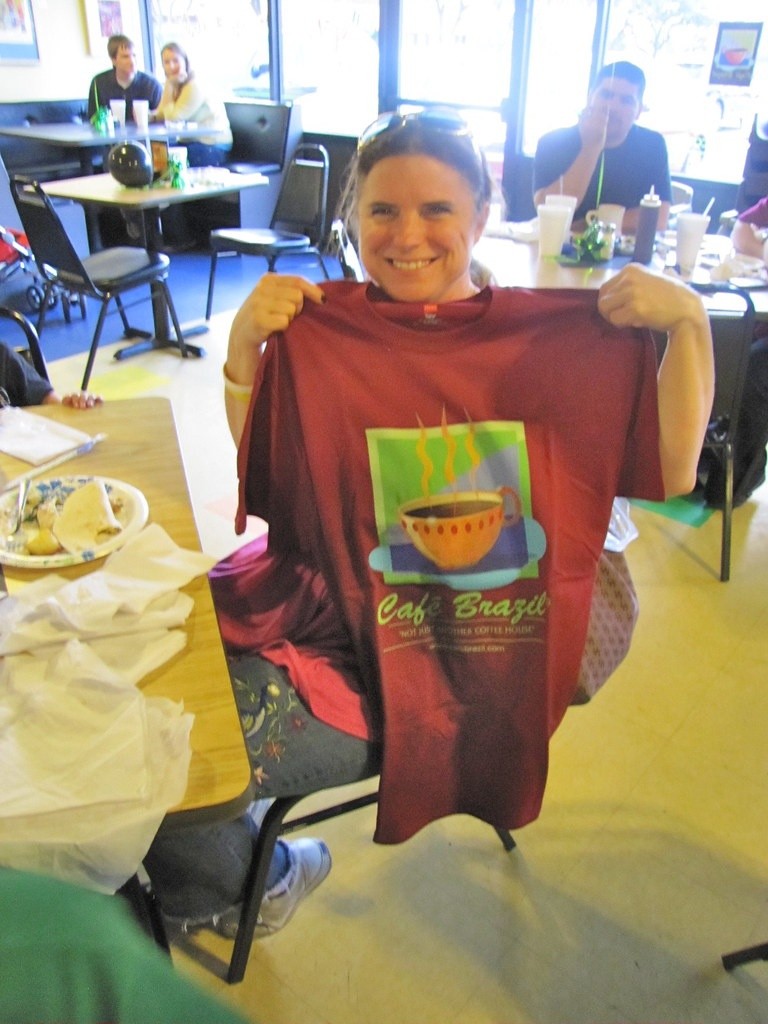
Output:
<box><xmin>167</xmin><ymin>154</ymin><xmax>185</xmax><ymax>189</ymax></box>
<box><xmin>595</xmin><ymin>222</ymin><xmax>618</xmax><ymax>260</ymax></box>
<box><xmin>633</xmin><ymin>185</ymin><xmax>661</xmax><ymax>266</ymax></box>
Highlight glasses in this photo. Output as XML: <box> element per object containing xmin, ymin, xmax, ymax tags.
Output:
<box><xmin>356</xmin><ymin>109</ymin><xmax>483</xmax><ymax>173</ymax></box>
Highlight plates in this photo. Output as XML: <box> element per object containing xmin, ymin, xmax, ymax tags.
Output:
<box><xmin>0</xmin><ymin>471</ymin><xmax>148</xmax><ymax>568</ymax></box>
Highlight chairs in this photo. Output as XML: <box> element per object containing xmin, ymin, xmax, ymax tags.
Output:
<box><xmin>0</xmin><ymin>305</ymin><xmax>48</xmax><ymax>382</ymax></box>
<box><xmin>227</xmin><ymin>774</ymin><xmax>517</xmax><ymax>985</ymax></box>
<box><xmin>205</xmin><ymin>143</ymin><xmax>329</xmax><ymax>321</ymax></box>
<box><xmin>330</xmin><ymin>219</ymin><xmax>363</xmax><ymax>282</ymax></box>
<box><xmin>656</xmin><ymin>284</ymin><xmax>757</xmax><ymax>582</ymax></box>
<box><xmin>9</xmin><ymin>174</ymin><xmax>189</xmax><ymax>390</ymax></box>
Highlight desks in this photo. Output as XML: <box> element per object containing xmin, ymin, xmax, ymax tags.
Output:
<box><xmin>21</xmin><ymin>166</ymin><xmax>268</xmax><ymax>360</ymax></box>
<box><xmin>0</xmin><ymin>122</ymin><xmax>224</xmax><ymax>253</ymax></box>
<box><xmin>470</xmin><ymin>231</ymin><xmax>768</xmax><ymax>469</ymax></box>
<box><xmin>0</xmin><ymin>396</ymin><xmax>254</xmax><ymax>963</ymax></box>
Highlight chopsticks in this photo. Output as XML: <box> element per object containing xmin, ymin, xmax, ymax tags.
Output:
<box><xmin>5</xmin><ymin>441</ymin><xmax>93</xmax><ymax>490</ymax></box>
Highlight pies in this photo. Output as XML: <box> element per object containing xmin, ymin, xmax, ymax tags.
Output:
<box><xmin>52</xmin><ymin>478</ymin><xmax>122</xmax><ymax>554</ymax></box>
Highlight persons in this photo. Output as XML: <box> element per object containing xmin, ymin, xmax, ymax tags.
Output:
<box><xmin>0</xmin><ymin>339</ymin><xmax>104</xmax><ymax>410</ymax></box>
<box><xmin>532</xmin><ymin>61</ymin><xmax>674</xmax><ymax>235</ymax></box>
<box><xmin>145</xmin><ymin>43</ymin><xmax>234</xmax><ymax>254</ymax></box>
<box><xmin>88</xmin><ymin>34</ymin><xmax>163</xmax><ymax>119</ymax></box>
<box><xmin>731</xmin><ymin>196</ymin><xmax>768</xmax><ymax>266</ymax></box>
<box><xmin>138</xmin><ymin>124</ymin><xmax>716</xmax><ymax>943</ymax></box>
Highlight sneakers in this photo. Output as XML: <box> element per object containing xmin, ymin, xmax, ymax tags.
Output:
<box><xmin>216</xmin><ymin>837</ymin><xmax>333</xmax><ymax>938</ymax></box>
<box><xmin>244</xmin><ymin>798</ymin><xmax>270</xmax><ymax>828</ymax></box>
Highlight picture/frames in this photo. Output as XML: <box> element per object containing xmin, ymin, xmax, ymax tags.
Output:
<box><xmin>1</xmin><ymin>0</ymin><xmax>43</xmax><ymax>68</ymax></box>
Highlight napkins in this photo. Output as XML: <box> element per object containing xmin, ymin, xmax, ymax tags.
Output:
<box><xmin>0</xmin><ymin>522</ymin><xmax>218</xmax><ymax>895</ymax></box>
<box><xmin>0</xmin><ymin>407</ymin><xmax>92</xmax><ymax>466</ymax></box>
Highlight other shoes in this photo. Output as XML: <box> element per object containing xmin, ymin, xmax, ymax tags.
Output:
<box><xmin>163</xmin><ymin>238</ymin><xmax>194</xmax><ymax>255</ymax></box>
<box><xmin>120</xmin><ymin>207</ymin><xmax>140</xmax><ymax>239</ymax></box>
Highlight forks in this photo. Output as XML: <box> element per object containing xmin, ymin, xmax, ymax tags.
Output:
<box><xmin>7</xmin><ymin>479</ymin><xmax>32</xmax><ymax>552</ymax></box>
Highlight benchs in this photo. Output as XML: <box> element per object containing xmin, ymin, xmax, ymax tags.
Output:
<box><xmin>0</xmin><ymin>100</ymin><xmax>105</xmax><ymax>183</ymax></box>
<box><xmin>196</xmin><ymin>102</ymin><xmax>302</xmax><ymax>258</ymax></box>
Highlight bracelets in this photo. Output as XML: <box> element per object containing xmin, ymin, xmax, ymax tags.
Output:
<box><xmin>223</xmin><ymin>361</ymin><xmax>254</xmax><ymax>394</ymax></box>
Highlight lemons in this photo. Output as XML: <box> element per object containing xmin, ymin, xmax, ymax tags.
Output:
<box><xmin>25</xmin><ymin>529</ymin><xmax>59</xmax><ymax>555</ymax></box>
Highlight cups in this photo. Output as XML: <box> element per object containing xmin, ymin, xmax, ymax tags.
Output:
<box><xmin>545</xmin><ymin>195</ymin><xmax>578</xmax><ymax>236</ymax></box>
<box><xmin>133</xmin><ymin>100</ymin><xmax>149</xmax><ymax>134</ymax></box>
<box><xmin>536</xmin><ymin>204</ymin><xmax>571</xmax><ymax>265</ymax></box>
<box><xmin>585</xmin><ymin>204</ymin><xmax>625</xmax><ymax>239</ymax></box>
<box><xmin>725</xmin><ymin>48</ymin><xmax>747</xmax><ymax>64</ymax></box>
<box><xmin>110</xmin><ymin>99</ymin><xmax>126</xmax><ymax>128</ymax></box>
<box><xmin>676</xmin><ymin>214</ymin><xmax>711</xmax><ymax>271</ymax></box>
